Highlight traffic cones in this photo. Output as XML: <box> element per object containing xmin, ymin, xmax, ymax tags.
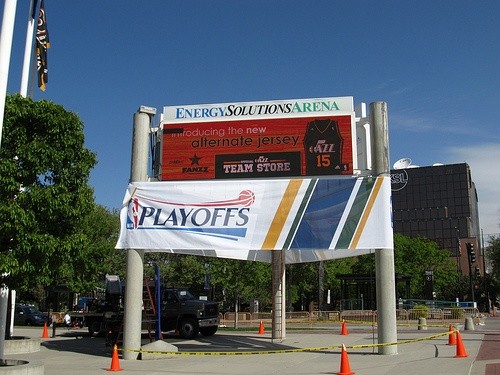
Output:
<box><xmin>340</xmin><ymin>321</ymin><xmax>350</xmax><ymax>335</ymax></box>
<box><xmin>257</xmin><ymin>319</ymin><xmax>265</xmax><ymax>335</ymax></box>
<box><xmin>445</xmin><ymin>324</ymin><xmax>457</xmax><ymax>345</ymax></box>
<box><xmin>452</xmin><ymin>331</ymin><xmax>468</xmax><ymax>358</ymax></box>
<box><xmin>40</xmin><ymin>322</ymin><xmax>49</xmax><ymax>338</ymax></box>
<box><xmin>105</xmin><ymin>343</ymin><xmax>125</xmax><ymax>372</ymax></box>
<box><xmin>336</xmin><ymin>343</ymin><xmax>356</xmax><ymax>375</ymax></box>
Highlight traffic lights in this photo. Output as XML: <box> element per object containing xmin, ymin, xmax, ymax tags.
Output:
<box><xmin>475</xmin><ymin>266</ymin><xmax>481</xmax><ymax>282</ymax></box>
<box><xmin>467</xmin><ymin>242</ymin><xmax>475</xmax><ymax>255</ymax></box>
<box><xmin>471</xmin><ymin>252</ymin><xmax>476</xmax><ymax>263</ymax></box>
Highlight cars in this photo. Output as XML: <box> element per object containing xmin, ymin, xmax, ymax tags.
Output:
<box><xmin>14</xmin><ymin>304</ymin><xmax>53</xmax><ymax>327</ymax></box>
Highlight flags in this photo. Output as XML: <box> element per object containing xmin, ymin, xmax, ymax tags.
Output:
<box><xmin>35</xmin><ymin>0</ymin><xmax>52</xmax><ymax>92</ymax></box>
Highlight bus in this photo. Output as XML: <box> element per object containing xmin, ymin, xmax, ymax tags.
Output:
<box><xmin>439</xmin><ymin>301</ymin><xmax>478</xmax><ymax>318</ymax></box>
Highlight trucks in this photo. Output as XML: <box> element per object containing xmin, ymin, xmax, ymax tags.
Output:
<box><xmin>68</xmin><ymin>278</ymin><xmax>219</xmax><ymax>340</ymax></box>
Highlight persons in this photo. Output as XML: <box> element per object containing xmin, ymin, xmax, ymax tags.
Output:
<box><xmin>64</xmin><ymin>312</ymin><xmax>71</xmax><ymax>330</ymax></box>
